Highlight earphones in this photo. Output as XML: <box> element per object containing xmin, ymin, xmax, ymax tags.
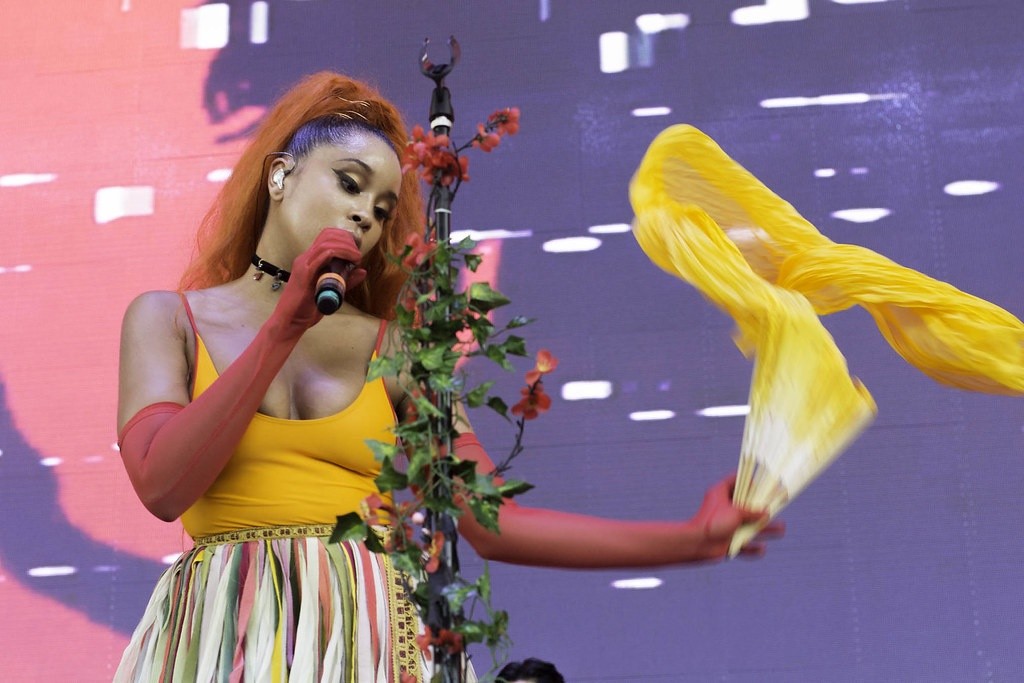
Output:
<box><xmin>271</xmin><ymin>169</ymin><xmax>291</xmax><ymax>189</ymax></box>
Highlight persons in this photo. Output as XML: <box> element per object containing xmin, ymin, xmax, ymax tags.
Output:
<box><xmin>495</xmin><ymin>657</ymin><xmax>564</xmax><ymax>682</ymax></box>
<box><xmin>111</xmin><ymin>65</ymin><xmax>786</xmax><ymax>683</ymax></box>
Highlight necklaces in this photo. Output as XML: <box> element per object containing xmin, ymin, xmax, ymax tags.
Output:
<box><xmin>252</xmin><ymin>252</ymin><xmax>290</xmax><ymax>290</ymax></box>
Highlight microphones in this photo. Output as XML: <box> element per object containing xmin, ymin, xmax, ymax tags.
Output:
<box><xmin>314</xmin><ymin>256</ymin><xmax>352</xmax><ymax>315</ymax></box>
